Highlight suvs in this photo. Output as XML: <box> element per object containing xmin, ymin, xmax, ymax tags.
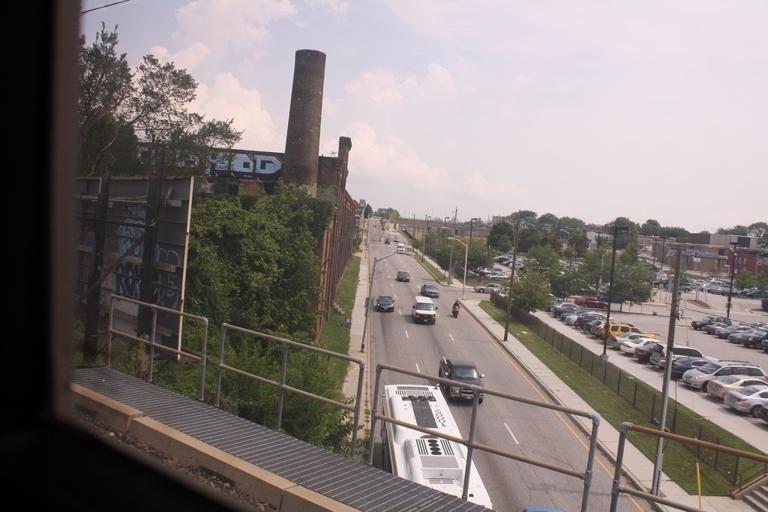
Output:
<box><xmin>439</xmin><ymin>354</ymin><xmax>486</xmax><ymax>405</ymax></box>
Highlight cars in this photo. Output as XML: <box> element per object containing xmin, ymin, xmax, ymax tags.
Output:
<box><xmin>637</xmin><ymin>252</ymin><xmax>661</xmax><ymax>264</ymax></box>
<box><xmin>421</xmin><ymin>284</ymin><xmax>439</xmax><ymax>298</ymax></box>
<box><xmin>472</xmin><ymin>254</ymin><xmax>526</xmax><ymax>294</ymax></box>
<box><xmin>385</xmin><ymin>239</ymin><xmax>391</xmax><ymax>244</ymax></box>
<box><xmin>397</xmin><ymin>270</ymin><xmax>410</xmax><ymax>282</ymax></box>
<box><xmin>550</xmin><ymin>270</ymin><xmax>768</xmax><ymax>422</ymax></box>
<box><xmin>376</xmin><ymin>295</ymin><xmax>395</xmax><ymax>313</ymax></box>
<box><xmin>394</xmin><ymin>238</ymin><xmax>400</xmax><ymax>242</ymax></box>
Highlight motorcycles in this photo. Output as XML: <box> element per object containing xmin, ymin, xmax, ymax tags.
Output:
<box><xmin>452</xmin><ymin>306</ymin><xmax>461</xmax><ymax>318</ymax></box>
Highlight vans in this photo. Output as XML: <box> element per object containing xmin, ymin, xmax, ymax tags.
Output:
<box><xmin>412</xmin><ymin>296</ymin><xmax>438</xmax><ymax>325</ymax></box>
<box><xmin>397</xmin><ymin>242</ymin><xmax>405</xmax><ymax>254</ymax></box>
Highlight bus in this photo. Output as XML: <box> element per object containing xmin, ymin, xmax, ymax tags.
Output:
<box><xmin>380</xmin><ymin>383</ymin><xmax>495</xmax><ymax>510</ymax></box>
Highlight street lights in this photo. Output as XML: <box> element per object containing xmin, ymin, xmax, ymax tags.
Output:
<box><xmin>445</xmin><ymin>216</ymin><xmax>449</xmax><ymax>227</ymax></box>
<box><xmin>470</xmin><ymin>218</ymin><xmax>481</xmax><ymax>248</ymax></box>
<box><xmin>726</xmin><ymin>242</ymin><xmax>736</xmax><ymax>317</ymax></box>
<box><xmin>439</xmin><ymin>226</ymin><xmax>454</xmax><ymax>282</ymax></box>
<box><xmin>357</xmin><ymin>250</ymin><xmax>416</xmax><ymax>354</ymax></box>
<box><xmin>602</xmin><ymin>227</ymin><xmax>630</xmax><ymax>357</ymax></box>
<box><xmin>427</xmin><ymin>216</ymin><xmax>432</xmax><ymax>227</ymax></box>
<box><xmin>503</xmin><ymin>211</ymin><xmax>537</xmax><ymax>343</ymax></box>
<box><xmin>446</xmin><ymin>236</ymin><xmax>468</xmax><ymax>300</ymax></box>
